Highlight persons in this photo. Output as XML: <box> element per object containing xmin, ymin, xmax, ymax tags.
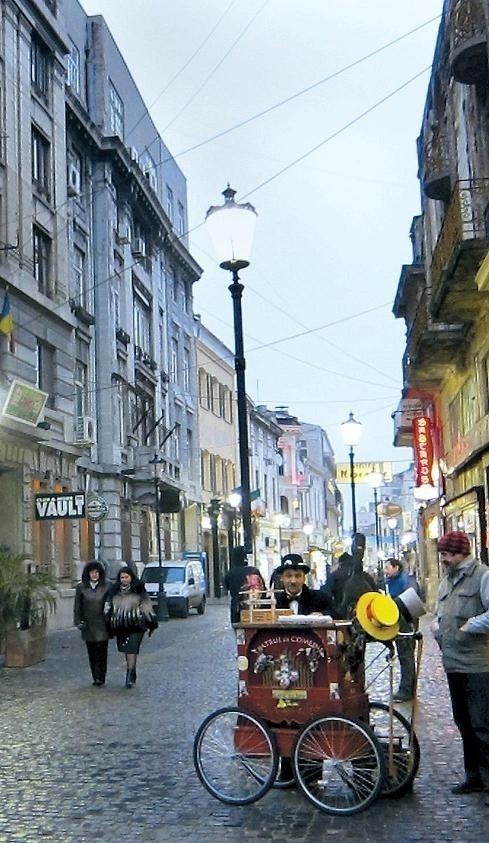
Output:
<box><xmin>258</xmin><ymin>553</ymin><xmax>333</xmax><ymax>786</ymax></box>
<box><xmin>104</xmin><ymin>566</ymin><xmax>157</xmax><ymax>687</ymax></box>
<box><xmin>71</xmin><ymin>563</ymin><xmax>112</xmax><ymax>687</ymax></box>
<box><xmin>324</xmin><ymin>552</ymin><xmax>381</xmax><ymax>689</ymax></box>
<box><xmin>378</xmin><ymin>557</ymin><xmax>423</xmax><ymax>704</ymax></box>
<box><xmin>430</xmin><ymin>532</ymin><xmax>489</xmax><ymax>800</ymax></box>
<box><xmin>223</xmin><ymin>546</ymin><xmax>266</xmax><ymax>629</ymax></box>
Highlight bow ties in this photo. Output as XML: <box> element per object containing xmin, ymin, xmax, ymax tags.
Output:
<box><xmin>288</xmin><ymin>596</ymin><xmax>301</xmax><ymax>603</ymax></box>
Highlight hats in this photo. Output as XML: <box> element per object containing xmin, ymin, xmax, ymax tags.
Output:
<box><xmin>276</xmin><ymin>554</ymin><xmax>310</xmax><ymax>575</ymax></box>
<box><xmin>356</xmin><ymin>587</ymin><xmax>427</xmax><ymax>641</ymax></box>
<box><xmin>438</xmin><ymin>531</ymin><xmax>469</xmax><ymax>555</ymax></box>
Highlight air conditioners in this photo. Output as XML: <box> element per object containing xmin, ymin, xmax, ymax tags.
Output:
<box><xmin>74</xmin><ymin>415</ymin><xmax>97</xmax><ymax>448</ymax></box>
<box><xmin>132</xmin><ymin>237</ymin><xmax>145</xmax><ymax>258</ymax></box>
<box><xmin>67</xmin><ymin>164</ymin><xmax>81</xmax><ymax>197</ymax></box>
<box><xmin>114</xmin><ymin>222</ymin><xmax>131</xmax><ymax>243</ymax></box>
<box><xmin>126</xmin><ymin>146</ymin><xmax>138</xmax><ymax>162</ymax></box>
<box><xmin>147</xmin><ymin>171</ymin><xmax>157</xmax><ymax>193</ymax></box>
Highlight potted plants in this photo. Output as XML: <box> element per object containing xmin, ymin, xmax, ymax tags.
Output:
<box><xmin>1</xmin><ymin>550</ymin><xmax>57</xmax><ymax>668</ymax></box>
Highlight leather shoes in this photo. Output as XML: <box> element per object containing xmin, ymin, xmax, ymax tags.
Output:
<box><xmin>96</xmin><ymin>679</ymin><xmax>102</xmax><ymax>686</ymax></box>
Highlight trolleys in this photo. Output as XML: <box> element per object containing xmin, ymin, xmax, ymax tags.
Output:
<box><xmin>192</xmin><ymin>620</ymin><xmax>424</xmax><ymax>817</ymax></box>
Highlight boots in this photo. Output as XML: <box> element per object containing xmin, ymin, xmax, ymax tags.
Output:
<box><xmin>126</xmin><ymin>669</ymin><xmax>136</xmax><ymax>688</ymax></box>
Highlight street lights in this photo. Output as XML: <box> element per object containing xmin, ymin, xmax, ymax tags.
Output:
<box><xmin>209</xmin><ymin>492</ymin><xmax>352</xmax><ymax>599</ymax></box>
<box><xmin>341</xmin><ymin>412</ymin><xmax>365</xmax><ymax>535</ymax></box>
<box><xmin>370</xmin><ymin>472</ymin><xmax>401</xmax><ymax>593</ymax></box>
<box><xmin>148</xmin><ymin>451</ymin><xmax>169</xmax><ymax>622</ymax></box>
<box><xmin>205</xmin><ymin>182</ymin><xmax>260</xmax><ymax>566</ymax></box>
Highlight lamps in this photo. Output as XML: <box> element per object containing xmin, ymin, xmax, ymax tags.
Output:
<box><xmin>456</xmin><ymin>516</ymin><xmax>465</xmax><ymax>529</ymax></box>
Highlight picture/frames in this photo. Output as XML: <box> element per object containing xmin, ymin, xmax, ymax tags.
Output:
<box><xmin>3</xmin><ymin>379</ymin><xmax>49</xmax><ymax>428</ymax></box>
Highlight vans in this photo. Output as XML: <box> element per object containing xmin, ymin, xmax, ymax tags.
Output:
<box><xmin>138</xmin><ymin>559</ymin><xmax>207</xmax><ymax>619</ymax></box>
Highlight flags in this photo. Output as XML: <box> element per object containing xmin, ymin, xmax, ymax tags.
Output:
<box><xmin>0</xmin><ymin>291</ymin><xmax>16</xmax><ymax>356</ymax></box>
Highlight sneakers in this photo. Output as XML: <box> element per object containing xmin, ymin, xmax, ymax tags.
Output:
<box><xmin>391</xmin><ymin>694</ymin><xmax>412</xmax><ymax>703</ymax></box>
<box><xmin>452</xmin><ymin>782</ymin><xmax>484</xmax><ymax>795</ymax></box>
